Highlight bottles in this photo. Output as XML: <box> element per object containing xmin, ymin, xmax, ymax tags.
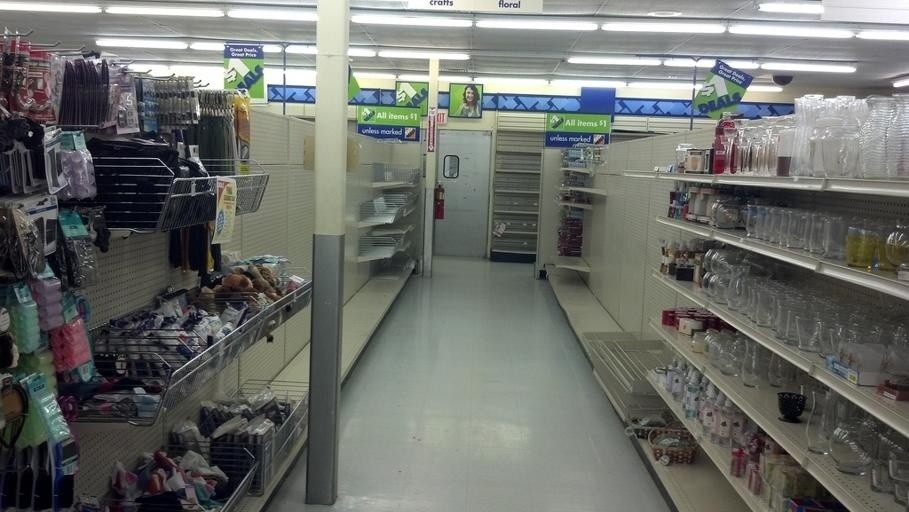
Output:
<box><xmin>660</xmin><ymin>93</ymin><xmax>908</xmax><ymax>511</ymax></box>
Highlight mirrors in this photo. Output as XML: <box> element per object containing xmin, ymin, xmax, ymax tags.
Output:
<box><xmin>443</xmin><ymin>155</ymin><xmax>459</xmax><ymax>178</ymax></box>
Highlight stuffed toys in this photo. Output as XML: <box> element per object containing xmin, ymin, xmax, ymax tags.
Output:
<box><xmin>186</xmin><ymin>263</ymin><xmax>294</xmax><ymax>342</ymax></box>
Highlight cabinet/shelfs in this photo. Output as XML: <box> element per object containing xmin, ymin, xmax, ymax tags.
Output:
<box><xmin>358</xmin><ymin>176</ymin><xmax>420</xmax><ymax>261</ymax></box>
<box><xmin>646</xmin><ymin>169</ymin><xmax>909</xmax><ymax>512</ymax></box>
<box><xmin>549</xmin><ymin>146</ymin><xmax>606</xmax><ymax>277</ymax></box>
<box><xmin>58</xmin><ymin>133</ymin><xmax>312</xmax><ymax>512</ymax></box>
<box><xmin>492</xmin><ymin>151</ymin><xmax>542</xmax><ymax>253</ymax></box>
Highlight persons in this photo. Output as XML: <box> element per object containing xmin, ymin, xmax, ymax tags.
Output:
<box><xmin>457</xmin><ymin>85</ymin><xmax>480</xmax><ymax>117</ymax></box>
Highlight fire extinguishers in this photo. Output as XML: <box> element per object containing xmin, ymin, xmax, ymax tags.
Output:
<box><xmin>434</xmin><ymin>183</ymin><xmax>445</xmax><ymax>219</ymax></box>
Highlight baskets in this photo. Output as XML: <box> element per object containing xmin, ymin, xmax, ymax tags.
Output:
<box><xmin>629</xmin><ymin>413</ymin><xmax>698</xmax><ymax>465</ymax></box>
